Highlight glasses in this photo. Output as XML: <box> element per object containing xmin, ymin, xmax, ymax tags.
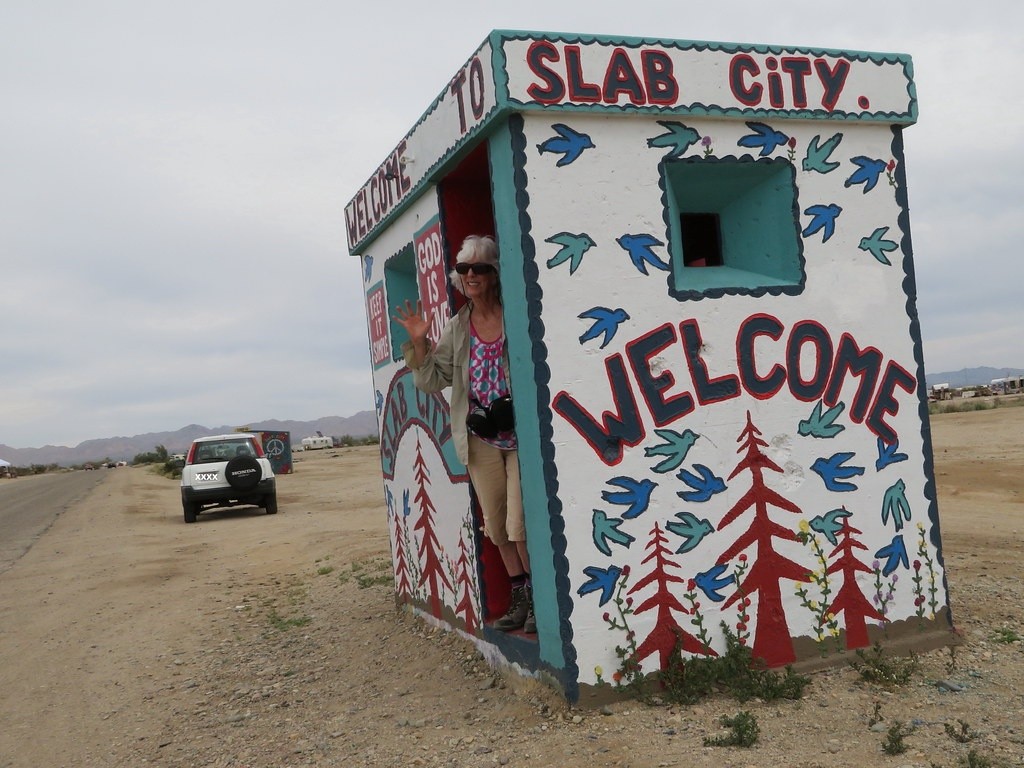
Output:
<box><xmin>455</xmin><ymin>262</ymin><xmax>497</xmax><ymax>275</ymax></box>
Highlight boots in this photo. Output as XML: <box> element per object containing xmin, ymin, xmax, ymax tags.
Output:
<box><xmin>494</xmin><ymin>585</ymin><xmax>528</xmax><ymax>630</ymax></box>
<box><xmin>523</xmin><ymin>584</ymin><xmax>537</xmax><ymax>632</ymax></box>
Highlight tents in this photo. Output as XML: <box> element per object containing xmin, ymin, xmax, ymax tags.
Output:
<box><xmin>0</xmin><ymin>459</ymin><xmax>11</xmax><ymax>474</ymax></box>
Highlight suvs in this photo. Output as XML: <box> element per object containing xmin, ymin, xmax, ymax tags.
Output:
<box><xmin>181</xmin><ymin>433</ymin><xmax>278</xmax><ymax>523</ymax></box>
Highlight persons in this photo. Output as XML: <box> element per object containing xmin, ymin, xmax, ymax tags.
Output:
<box><xmin>392</xmin><ymin>234</ymin><xmax>538</xmax><ymax>636</ymax></box>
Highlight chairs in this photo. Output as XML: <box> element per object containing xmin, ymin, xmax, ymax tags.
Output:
<box><xmin>236</xmin><ymin>445</ymin><xmax>251</xmax><ymax>458</ymax></box>
<box><xmin>200</xmin><ymin>450</ymin><xmax>213</xmax><ymax>459</ymax></box>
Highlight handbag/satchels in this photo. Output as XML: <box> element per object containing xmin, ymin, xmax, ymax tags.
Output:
<box><xmin>466</xmin><ymin>395</ymin><xmax>515</xmax><ymax>439</ymax></box>
